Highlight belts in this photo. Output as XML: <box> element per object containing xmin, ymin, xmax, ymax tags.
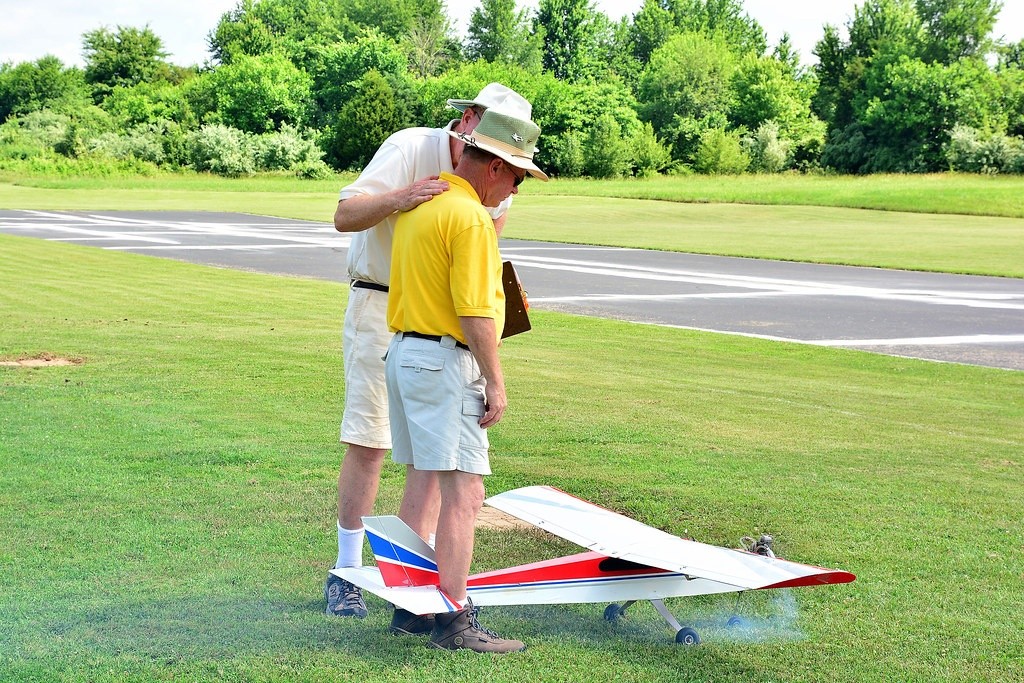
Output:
<box><xmin>350</xmin><ymin>278</ymin><xmax>389</xmax><ymax>292</ymax></box>
<box><xmin>396</xmin><ymin>331</ymin><xmax>471</xmax><ymax>352</ymax></box>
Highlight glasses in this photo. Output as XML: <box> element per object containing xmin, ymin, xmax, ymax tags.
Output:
<box><xmin>502</xmin><ymin>160</ymin><xmax>524</xmax><ymax>187</ymax></box>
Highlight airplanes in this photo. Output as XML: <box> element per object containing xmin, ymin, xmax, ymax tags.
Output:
<box><xmin>327</xmin><ymin>485</ymin><xmax>858</xmax><ymax>646</ymax></box>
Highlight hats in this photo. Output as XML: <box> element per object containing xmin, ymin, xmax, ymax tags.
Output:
<box><xmin>446</xmin><ymin>108</ymin><xmax>549</xmax><ymax>182</ymax></box>
<box><xmin>447</xmin><ymin>82</ymin><xmax>540</xmax><ymax>152</ymax></box>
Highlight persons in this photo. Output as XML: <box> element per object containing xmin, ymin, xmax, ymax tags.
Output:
<box><xmin>322</xmin><ymin>82</ymin><xmax>533</xmax><ymax>618</ymax></box>
<box><xmin>382</xmin><ymin>108</ymin><xmax>551</xmax><ymax>654</ymax></box>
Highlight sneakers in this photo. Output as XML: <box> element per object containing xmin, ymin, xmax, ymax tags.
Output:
<box><xmin>428</xmin><ymin>596</ymin><xmax>525</xmax><ymax>653</ymax></box>
<box><xmin>323</xmin><ymin>567</ymin><xmax>369</xmax><ymax>620</ymax></box>
<box><xmin>390</xmin><ymin>607</ymin><xmax>436</xmax><ymax>636</ymax></box>
